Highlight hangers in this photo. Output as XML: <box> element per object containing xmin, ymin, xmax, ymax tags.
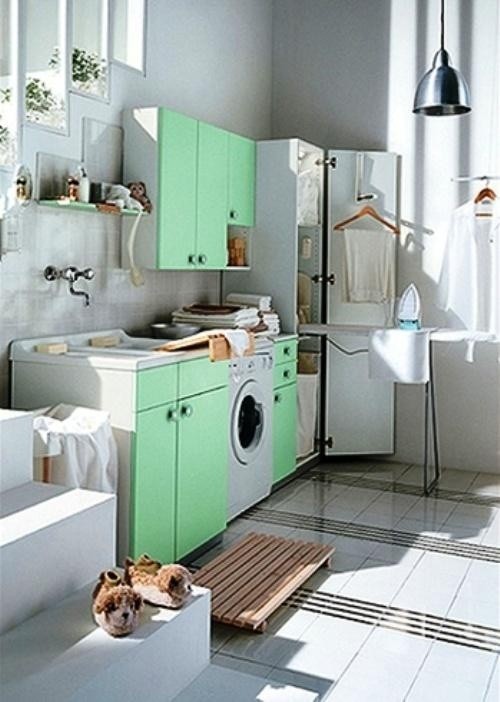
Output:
<box><xmin>331</xmin><ymin>194</ymin><xmax>399</xmax><ymax>236</ymax></box>
<box><xmin>471</xmin><ymin>173</ymin><xmax>497</xmax><ymax>218</ymax></box>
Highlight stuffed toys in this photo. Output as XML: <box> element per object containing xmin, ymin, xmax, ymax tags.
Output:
<box><xmin>127</xmin><ymin>181</ymin><xmax>151</xmax><ymax>213</ymax></box>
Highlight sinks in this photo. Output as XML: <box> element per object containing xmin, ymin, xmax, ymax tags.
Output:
<box><xmin>30</xmin><ymin>338</ymin><xmax>211</xmax><ymax>372</ymax></box>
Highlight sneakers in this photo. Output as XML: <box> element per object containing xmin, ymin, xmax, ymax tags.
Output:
<box><xmin>92</xmin><ymin>570</ymin><xmax>143</xmax><ymax>637</ymax></box>
<box><xmin>129</xmin><ymin>554</ymin><xmax>191</xmax><ymax>608</ymax></box>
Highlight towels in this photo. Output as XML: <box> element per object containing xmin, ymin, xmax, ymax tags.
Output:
<box><xmin>342</xmin><ymin>228</ymin><xmax>394</xmax><ymax>307</ymax></box>
<box><xmin>365</xmin><ymin>324</ymin><xmax>435</xmax><ymax>386</ymax></box>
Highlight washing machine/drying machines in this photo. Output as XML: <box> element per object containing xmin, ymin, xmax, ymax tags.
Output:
<box><xmin>228</xmin><ymin>341</ymin><xmax>273</xmax><ymax>525</ymax></box>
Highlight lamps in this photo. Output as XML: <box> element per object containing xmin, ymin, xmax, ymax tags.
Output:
<box><xmin>412</xmin><ymin>1</ymin><xmax>474</xmax><ymax>117</ymax></box>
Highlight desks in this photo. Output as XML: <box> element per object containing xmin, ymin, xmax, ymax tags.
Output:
<box><xmin>297</xmin><ymin>324</ymin><xmax>499</xmax><ymax>499</ymax></box>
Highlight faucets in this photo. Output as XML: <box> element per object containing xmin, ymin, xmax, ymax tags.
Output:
<box><xmin>69</xmin><ymin>281</ymin><xmax>90</xmax><ymax>307</ymax></box>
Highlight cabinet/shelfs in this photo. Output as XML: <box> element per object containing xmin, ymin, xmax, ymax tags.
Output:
<box><xmin>271</xmin><ymin>342</ymin><xmax>299</xmax><ymax>484</ymax></box>
<box><xmin>8</xmin><ymin>361</ymin><xmax>230</xmax><ymax>571</ymax></box>
<box><xmin>122</xmin><ymin>110</ymin><xmax>228</xmax><ymax>274</ymax></box>
<box><xmin>226</xmin><ymin>131</ymin><xmax>256</xmax><ymax>269</ymax></box>
<box><xmin>225</xmin><ymin>135</ymin><xmax>399</xmax><ymax>467</ymax></box>
